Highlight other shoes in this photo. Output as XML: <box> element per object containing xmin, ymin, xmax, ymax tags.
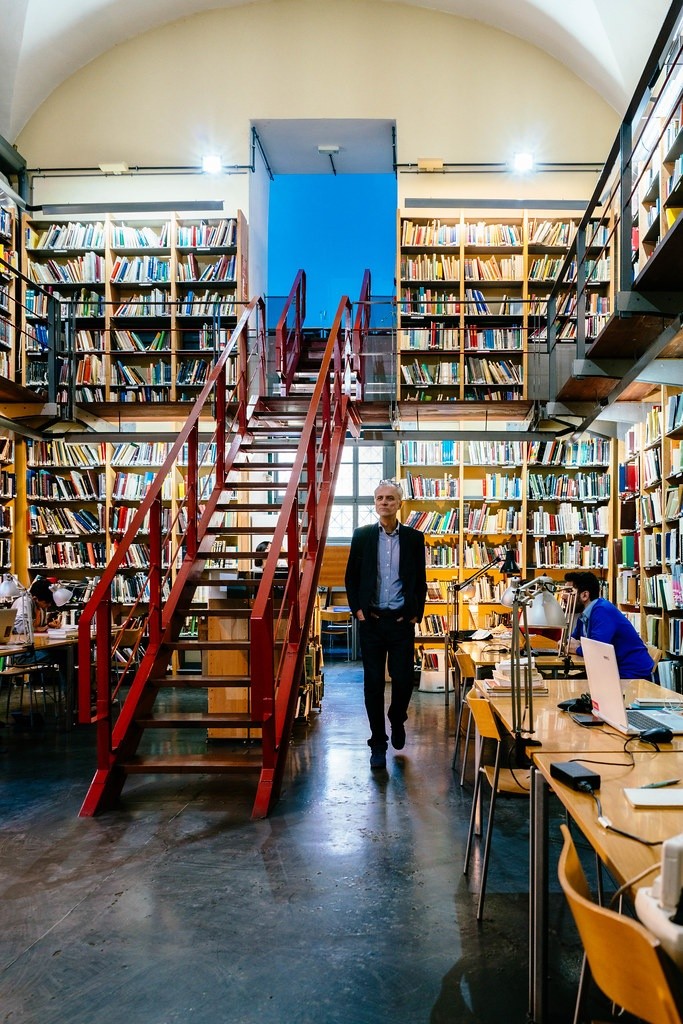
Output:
<box><xmin>370</xmin><ymin>746</ymin><xmax>386</xmax><ymax>770</ymax></box>
<box><xmin>390</xmin><ymin>722</ymin><xmax>406</xmax><ymax>750</ymax></box>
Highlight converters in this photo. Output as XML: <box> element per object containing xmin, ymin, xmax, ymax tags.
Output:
<box><xmin>549</xmin><ymin>762</ymin><xmax>601</xmax><ymax>792</ymax></box>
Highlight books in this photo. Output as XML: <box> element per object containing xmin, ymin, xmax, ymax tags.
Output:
<box><xmin>632</xmin><ymin>104</ymin><xmax>683</xmax><ymax>282</ymax></box>
<box><xmin>399</xmin><ymin>220</ymin><xmax>610</xmax><ymax>401</ymax></box>
<box><xmin>624</xmin><ymin>788</ymin><xmax>683</xmax><ymax>810</ymax></box>
<box><xmin>400</xmin><ymin>392</ymin><xmax>683</xmax><ymax>697</ymax></box>
<box><xmin>0</xmin><ymin>208</ymin><xmax>238</xmax><ymax>403</ymax></box>
<box><xmin>0</xmin><ymin>435</ymin><xmax>237</xmax><ymax>664</ymax></box>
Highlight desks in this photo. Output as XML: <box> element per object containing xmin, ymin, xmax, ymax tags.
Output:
<box><xmin>472</xmin><ymin>678</ymin><xmax>683</xmax><ymax>1019</ymax></box>
<box><xmin>0</xmin><ymin>635</ymin><xmax>77</xmax><ymax>735</ymax></box>
<box><xmin>532</xmin><ymin>752</ymin><xmax>683</xmax><ymax>1024</ymax></box>
<box><xmin>455</xmin><ymin>642</ymin><xmax>585</xmax><ymax>783</ymax></box>
<box><xmin>444</xmin><ymin>635</ymin><xmax>555</xmax><ymax>717</ymax></box>
<box><xmin>19</xmin><ymin>628</ymin><xmax>128</xmax><ymax>720</ymax></box>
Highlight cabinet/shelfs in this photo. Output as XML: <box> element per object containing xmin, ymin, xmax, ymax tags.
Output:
<box><xmin>0</xmin><ymin>18</ymin><xmax>683</xmax><ymax>695</ymax></box>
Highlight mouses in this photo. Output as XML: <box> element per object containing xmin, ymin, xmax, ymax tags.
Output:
<box><xmin>641</xmin><ymin>728</ymin><xmax>673</xmax><ymax>742</ymax></box>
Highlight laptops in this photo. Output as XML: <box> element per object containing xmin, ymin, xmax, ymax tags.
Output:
<box><xmin>509</xmin><ymin>620</ymin><xmax>563</xmax><ymax>656</ymax></box>
<box><xmin>0</xmin><ymin>609</ymin><xmax>17</xmax><ymax>644</ymax></box>
<box><xmin>580</xmin><ymin>637</ymin><xmax>683</xmax><ymax>736</ymax></box>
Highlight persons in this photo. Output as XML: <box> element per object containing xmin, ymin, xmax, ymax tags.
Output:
<box><xmin>12</xmin><ymin>578</ymin><xmax>53</xmax><ymax>665</ymax></box>
<box><xmin>562</xmin><ymin>572</ymin><xmax>655</xmax><ymax>679</ymax></box>
<box><xmin>344</xmin><ymin>482</ymin><xmax>428</xmax><ymax>751</ymax></box>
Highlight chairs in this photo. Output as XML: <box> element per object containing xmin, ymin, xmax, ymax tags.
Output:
<box><xmin>0</xmin><ymin>587</ymin><xmax>683</xmax><ymax>1024</ymax></box>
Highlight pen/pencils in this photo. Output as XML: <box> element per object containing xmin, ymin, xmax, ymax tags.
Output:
<box><xmin>52</xmin><ymin>618</ymin><xmax>59</xmax><ymax>629</ymax></box>
<box><xmin>642</xmin><ymin>780</ymin><xmax>680</xmax><ymax>788</ymax></box>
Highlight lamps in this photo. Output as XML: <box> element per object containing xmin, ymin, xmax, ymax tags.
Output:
<box><xmin>417</xmin><ymin>158</ymin><xmax>444</xmax><ymax>172</ymax></box>
<box><xmin>449</xmin><ymin>550</ymin><xmax>520</xmax><ymax>639</ymax></box>
<box><xmin>97</xmin><ymin>162</ymin><xmax>129</xmax><ymax>176</ymax></box>
<box><xmin>316</xmin><ymin>144</ymin><xmax>339</xmax><ymax>155</ymax></box>
<box><xmin>501</xmin><ymin>575</ymin><xmax>565</xmax><ymax>750</ymax></box>
